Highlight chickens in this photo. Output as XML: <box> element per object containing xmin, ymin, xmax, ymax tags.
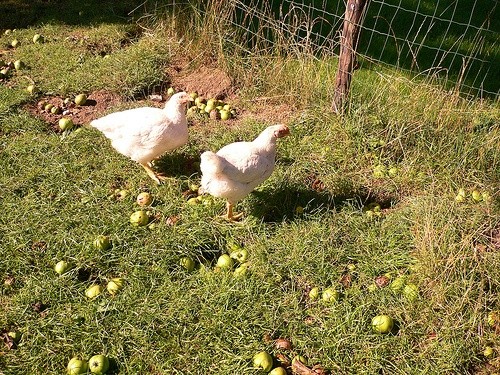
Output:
<box><xmin>200</xmin><ymin>124</ymin><xmax>292</xmax><ymax>222</ymax></box>
<box><xmin>89</xmin><ymin>92</ymin><xmax>193</xmax><ymax>186</ymax></box>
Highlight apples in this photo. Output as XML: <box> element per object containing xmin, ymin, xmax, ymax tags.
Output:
<box><xmin>0</xmin><ymin>11</ymin><xmax>497</xmax><ymax>375</ymax></box>
